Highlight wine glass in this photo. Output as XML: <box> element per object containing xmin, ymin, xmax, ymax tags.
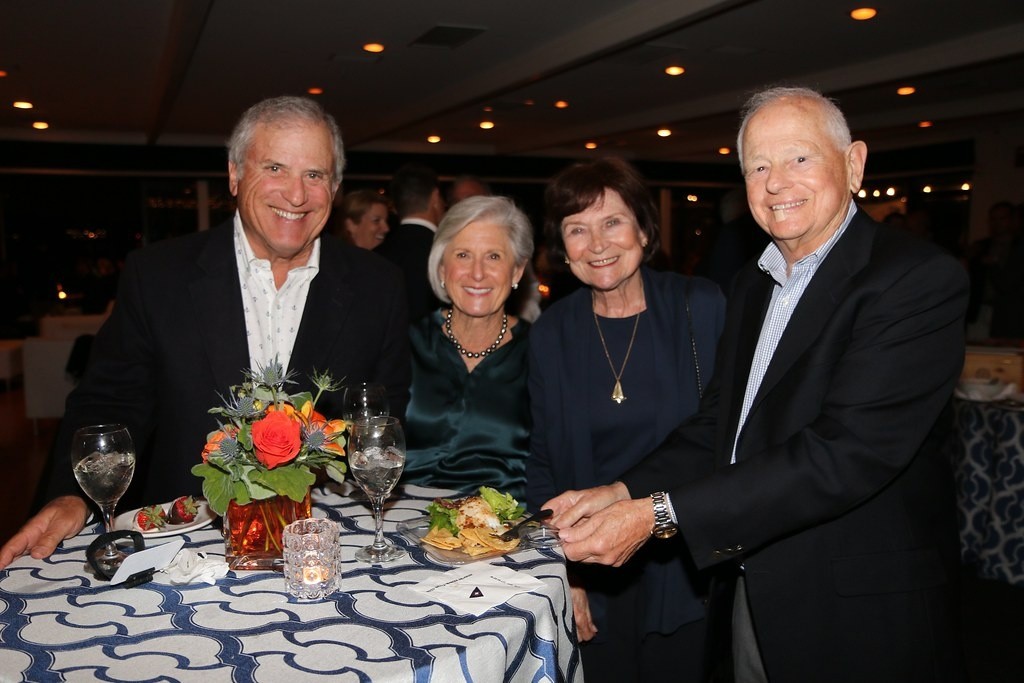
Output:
<box><xmin>348</xmin><ymin>416</ymin><xmax>407</xmax><ymax>563</ymax></box>
<box><xmin>70</xmin><ymin>424</ymin><xmax>136</xmax><ymax>574</ymax></box>
<box><xmin>342</xmin><ymin>379</ymin><xmax>391</xmax><ymax>500</ymax></box>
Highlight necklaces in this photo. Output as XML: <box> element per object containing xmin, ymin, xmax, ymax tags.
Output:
<box><xmin>445</xmin><ymin>309</ymin><xmax>505</xmax><ymax>357</ymax></box>
<box><xmin>593</xmin><ymin>311</ymin><xmax>640</xmax><ymax>403</ymax></box>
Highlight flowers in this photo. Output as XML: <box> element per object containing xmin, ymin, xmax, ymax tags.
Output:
<box><xmin>191</xmin><ymin>353</ymin><xmax>347</xmax><ymax>516</ymax></box>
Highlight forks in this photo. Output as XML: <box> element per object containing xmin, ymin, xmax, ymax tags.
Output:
<box><xmin>489</xmin><ymin>508</ymin><xmax>553</xmax><ymax>541</ymax></box>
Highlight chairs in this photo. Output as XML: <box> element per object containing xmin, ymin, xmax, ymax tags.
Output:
<box><xmin>0</xmin><ymin>339</ymin><xmax>23</xmax><ymax>391</ymax></box>
<box><xmin>23</xmin><ymin>337</ymin><xmax>74</xmax><ymax>436</ymax></box>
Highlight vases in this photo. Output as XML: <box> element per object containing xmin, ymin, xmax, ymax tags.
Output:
<box><xmin>222</xmin><ymin>485</ymin><xmax>312</xmax><ymax>570</ymax></box>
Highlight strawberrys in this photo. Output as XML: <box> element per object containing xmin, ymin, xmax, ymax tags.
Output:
<box><xmin>136</xmin><ymin>494</ymin><xmax>201</xmax><ymax>531</ymax></box>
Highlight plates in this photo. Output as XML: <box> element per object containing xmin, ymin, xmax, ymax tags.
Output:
<box><xmin>112</xmin><ymin>500</ymin><xmax>217</xmax><ymax>538</ymax></box>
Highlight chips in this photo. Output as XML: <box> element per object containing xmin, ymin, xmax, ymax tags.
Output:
<box><xmin>420</xmin><ymin>527</ymin><xmax>520</xmax><ymax>557</ymax></box>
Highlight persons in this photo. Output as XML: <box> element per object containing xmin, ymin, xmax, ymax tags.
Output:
<box><xmin>448</xmin><ymin>172</ymin><xmax>488</xmax><ymax>208</ymax></box>
<box><xmin>337</xmin><ymin>187</ymin><xmax>390</xmax><ymax>250</ymax></box>
<box><xmin>372</xmin><ymin>160</ymin><xmax>451</xmax><ymax>326</ymax></box>
<box><xmin>374</xmin><ymin>195</ymin><xmax>535</xmax><ymax>500</ymax></box>
<box><xmin>0</xmin><ymin>95</ymin><xmax>408</xmax><ymax>571</ymax></box>
<box><xmin>884</xmin><ymin>200</ymin><xmax>1024</xmax><ymax>341</ymax></box>
<box><xmin>539</xmin><ymin>89</ymin><xmax>966</xmax><ymax>683</ymax></box>
<box><xmin>526</xmin><ymin>154</ymin><xmax>730</xmax><ymax>683</ymax></box>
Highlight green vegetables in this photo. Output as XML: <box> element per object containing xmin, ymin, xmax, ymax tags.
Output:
<box><xmin>425</xmin><ymin>485</ymin><xmax>526</xmax><ymax>536</ymax></box>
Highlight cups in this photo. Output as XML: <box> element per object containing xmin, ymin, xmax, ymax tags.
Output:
<box><xmin>282</xmin><ymin>516</ymin><xmax>341</xmax><ymax>599</ymax></box>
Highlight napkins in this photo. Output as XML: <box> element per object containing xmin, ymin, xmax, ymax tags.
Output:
<box><xmin>408</xmin><ymin>560</ymin><xmax>547</xmax><ymax>616</ymax></box>
<box><xmin>160</xmin><ymin>548</ymin><xmax>230</xmax><ymax>585</ymax></box>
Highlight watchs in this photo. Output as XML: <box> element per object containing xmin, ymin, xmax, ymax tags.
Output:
<box><xmin>650</xmin><ymin>492</ymin><xmax>679</xmax><ymax>538</ymax></box>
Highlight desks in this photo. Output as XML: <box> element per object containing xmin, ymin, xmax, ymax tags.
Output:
<box><xmin>950</xmin><ymin>396</ymin><xmax>1024</xmax><ymax>587</ymax></box>
<box><xmin>0</xmin><ymin>480</ymin><xmax>584</xmax><ymax>683</ymax></box>
<box><xmin>40</xmin><ymin>316</ymin><xmax>103</xmax><ymax>338</ymax></box>
